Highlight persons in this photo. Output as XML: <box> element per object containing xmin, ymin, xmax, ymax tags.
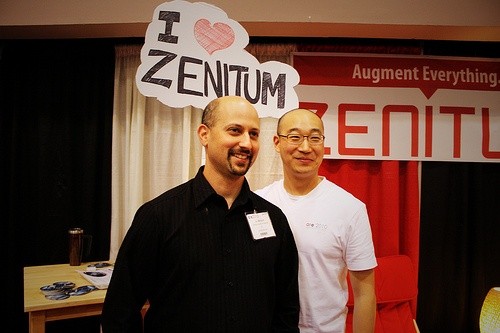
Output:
<box><xmin>253</xmin><ymin>108</ymin><xmax>378</xmax><ymax>333</ymax></box>
<box><xmin>99</xmin><ymin>95</ymin><xmax>301</xmax><ymax>333</ymax></box>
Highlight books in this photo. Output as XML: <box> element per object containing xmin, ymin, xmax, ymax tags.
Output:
<box><xmin>76</xmin><ymin>267</ymin><xmax>114</xmax><ymax>290</ymax></box>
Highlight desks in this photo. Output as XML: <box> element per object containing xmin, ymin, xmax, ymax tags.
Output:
<box><xmin>23</xmin><ymin>260</ymin><xmax>150</xmax><ymax>333</ymax></box>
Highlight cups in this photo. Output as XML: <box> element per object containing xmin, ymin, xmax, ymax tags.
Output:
<box><xmin>70</xmin><ymin>228</ymin><xmax>83</xmax><ymax>267</ymax></box>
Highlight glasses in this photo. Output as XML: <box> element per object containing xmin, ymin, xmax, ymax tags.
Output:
<box><xmin>278</xmin><ymin>133</ymin><xmax>325</xmax><ymax>144</ymax></box>
